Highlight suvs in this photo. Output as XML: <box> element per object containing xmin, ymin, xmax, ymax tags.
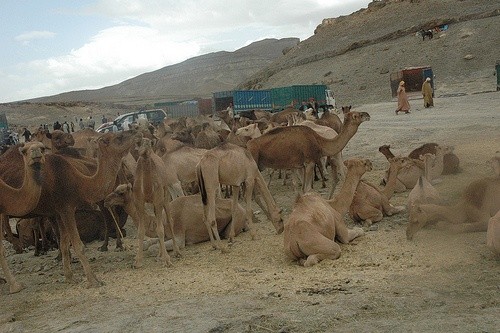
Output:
<box><xmin>95</xmin><ymin>109</ymin><xmax>168</xmax><ymax>135</ymax></box>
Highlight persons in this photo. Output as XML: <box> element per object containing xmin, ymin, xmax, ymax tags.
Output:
<box><xmin>299</xmin><ymin>96</ymin><xmax>338</xmax><ymax>116</ymax></box>
<box><xmin>394</xmin><ymin>80</ymin><xmax>411</xmax><ymax>115</ymax></box>
<box><xmin>415</xmin><ymin>81</ymin><xmax>420</xmax><ymax>90</ymax></box>
<box><xmin>116</xmin><ymin>113</ymin><xmax>120</xmax><ymax>116</ymax></box>
<box><xmin>79</xmin><ymin>119</ymin><xmax>84</xmax><ymax>129</ymax></box>
<box><xmin>414</xmin><ymin>25</ymin><xmax>441</xmax><ymax>39</ymax></box>
<box><xmin>88</xmin><ymin>117</ymin><xmax>95</xmax><ymax>128</ymax></box>
<box><xmin>22</xmin><ymin>127</ymin><xmax>31</xmax><ymax>142</ymax></box>
<box><xmin>38</xmin><ymin>123</ymin><xmax>48</xmax><ymax>130</ymax></box>
<box><xmin>0</xmin><ymin>124</ymin><xmax>19</xmax><ymax>146</ymax></box>
<box><xmin>493</xmin><ymin>60</ymin><xmax>500</xmax><ymax>84</ymax></box>
<box><xmin>53</xmin><ymin>121</ymin><xmax>63</xmax><ymax>129</ymax></box>
<box><xmin>226</xmin><ymin>102</ymin><xmax>233</xmax><ymax>116</ymax></box>
<box><xmin>421</xmin><ymin>77</ymin><xmax>435</xmax><ymax>108</ymax></box>
<box><xmin>102</xmin><ymin>115</ymin><xmax>107</xmax><ymax>123</ymax></box>
<box><xmin>71</xmin><ymin>121</ymin><xmax>75</xmax><ymax>132</ymax></box>
<box><xmin>63</xmin><ymin>121</ymin><xmax>70</xmax><ymax>133</ymax></box>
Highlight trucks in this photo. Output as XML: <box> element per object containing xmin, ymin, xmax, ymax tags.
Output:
<box><xmin>212</xmin><ymin>90</ymin><xmax>272</xmax><ymax>115</ymax></box>
<box><xmin>271</xmin><ymin>83</ymin><xmax>336</xmax><ymax>114</ymax></box>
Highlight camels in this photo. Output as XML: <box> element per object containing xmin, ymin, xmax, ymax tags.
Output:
<box><xmin>0</xmin><ymin>103</ymin><xmax>500</xmax><ymax>294</ymax></box>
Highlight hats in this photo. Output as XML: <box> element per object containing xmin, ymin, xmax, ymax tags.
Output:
<box><xmin>400</xmin><ymin>81</ymin><xmax>405</xmax><ymax>85</ymax></box>
<box><xmin>309</xmin><ymin>98</ymin><xmax>315</xmax><ymax>101</ymax></box>
<box><xmin>426</xmin><ymin>77</ymin><xmax>431</xmax><ymax>82</ymax></box>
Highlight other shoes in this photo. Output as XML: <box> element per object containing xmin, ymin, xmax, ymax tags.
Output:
<box><xmin>405</xmin><ymin>111</ymin><xmax>409</xmax><ymax>114</ymax></box>
<box><xmin>395</xmin><ymin>110</ymin><xmax>399</xmax><ymax>115</ymax></box>
